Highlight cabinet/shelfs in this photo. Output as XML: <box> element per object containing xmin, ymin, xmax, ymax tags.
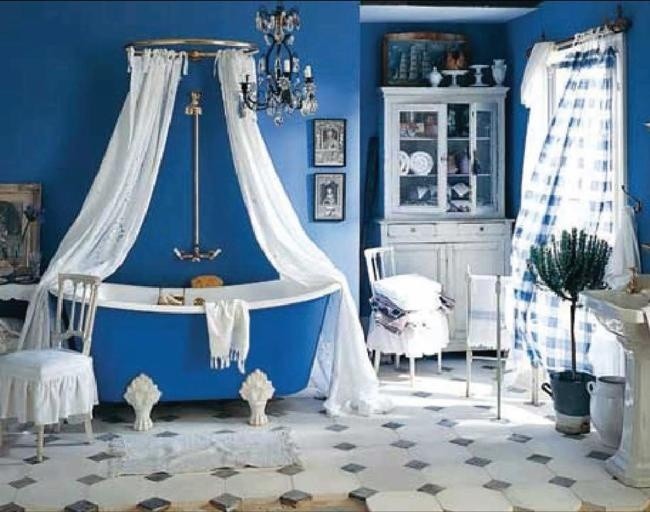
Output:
<box><xmin>370</xmin><ymin>215</ymin><xmax>516</xmax><ymax>362</ymax></box>
<box><xmin>373</xmin><ymin>85</ymin><xmax>510</xmax><ymax>218</ymax></box>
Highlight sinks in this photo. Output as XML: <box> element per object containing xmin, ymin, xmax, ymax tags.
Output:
<box><xmin>579</xmin><ymin>274</ymin><xmax>649</xmax><ymax>349</ymax></box>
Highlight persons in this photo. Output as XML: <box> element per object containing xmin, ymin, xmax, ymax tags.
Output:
<box><xmin>321</xmin><ymin>186</ymin><xmax>336</xmax><ymax>207</ymax></box>
<box><xmin>322</xmin><ymin>130</ymin><xmax>338</xmax><ymax>149</ymax></box>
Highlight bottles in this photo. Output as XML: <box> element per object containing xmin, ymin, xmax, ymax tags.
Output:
<box><xmin>428</xmin><ymin>64</ymin><xmax>443</xmax><ymax>87</ymax></box>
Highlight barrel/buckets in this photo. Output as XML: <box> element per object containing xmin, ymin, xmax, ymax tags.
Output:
<box><xmin>48</xmin><ymin>276</ymin><xmax>341</xmax><ymax>402</ymax></box>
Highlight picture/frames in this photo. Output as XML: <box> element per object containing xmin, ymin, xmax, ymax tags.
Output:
<box><xmin>0</xmin><ymin>181</ymin><xmax>43</xmax><ymax>276</ymax></box>
<box><xmin>311</xmin><ymin>117</ymin><xmax>346</xmax><ymax>222</ymax></box>
<box><xmin>381</xmin><ymin>31</ymin><xmax>473</xmax><ymax>86</ymax></box>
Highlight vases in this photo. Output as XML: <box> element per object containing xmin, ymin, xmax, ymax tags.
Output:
<box><xmin>4</xmin><ymin>234</ymin><xmax>29</xmax><ymax>283</ymax></box>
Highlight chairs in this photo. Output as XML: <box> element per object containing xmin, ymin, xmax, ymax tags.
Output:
<box><xmin>363</xmin><ymin>246</ymin><xmax>540</xmax><ymax>420</ymax></box>
<box><xmin>0</xmin><ymin>272</ymin><xmax>102</xmax><ymax>462</ymax></box>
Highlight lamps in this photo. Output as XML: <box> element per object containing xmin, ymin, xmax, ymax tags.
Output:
<box><xmin>233</xmin><ymin>2</ymin><xmax>319</xmax><ymax>128</ymax></box>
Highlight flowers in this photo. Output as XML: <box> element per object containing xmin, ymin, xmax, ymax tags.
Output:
<box><xmin>1</xmin><ymin>200</ymin><xmax>41</xmax><ymax>270</ymax></box>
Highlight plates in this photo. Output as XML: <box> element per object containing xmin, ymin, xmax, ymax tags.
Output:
<box><xmin>400</xmin><ymin>150</ymin><xmax>434</xmax><ymax>176</ymax></box>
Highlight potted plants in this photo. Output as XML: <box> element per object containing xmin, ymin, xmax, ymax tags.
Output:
<box><xmin>526</xmin><ymin>226</ymin><xmax>614</xmax><ymax>437</ymax></box>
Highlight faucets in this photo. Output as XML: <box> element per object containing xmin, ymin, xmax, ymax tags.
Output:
<box><xmin>624</xmin><ymin>264</ymin><xmax>644</xmax><ymax>294</ymax></box>
<box><xmin>192</xmin><ymin>245</ymin><xmax>202</xmax><ymax>263</ymax></box>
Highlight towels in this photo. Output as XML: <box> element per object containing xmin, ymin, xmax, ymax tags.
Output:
<box><xmin>107</xmin><ymin>428</ymin><xmax>304</xmax><ymax>480</ymax></box>
